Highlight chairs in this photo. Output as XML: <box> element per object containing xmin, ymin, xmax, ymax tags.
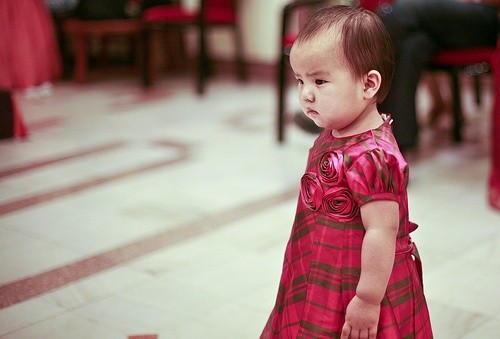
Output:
<box><xmin>279</xmin><ymin>1</ymin><xmax>499</xmax><ymax>209</ymax></box>
<box><xmin>141</xmin><ymin>1</ymin><xmax>247</xmax><ymax>95</ymax></box>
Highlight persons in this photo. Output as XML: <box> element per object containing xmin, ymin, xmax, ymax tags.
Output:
<box><xmin>258</xmin><ymin>6</ymin><xmax>435</xmax><ymax>339</ymax></box>
